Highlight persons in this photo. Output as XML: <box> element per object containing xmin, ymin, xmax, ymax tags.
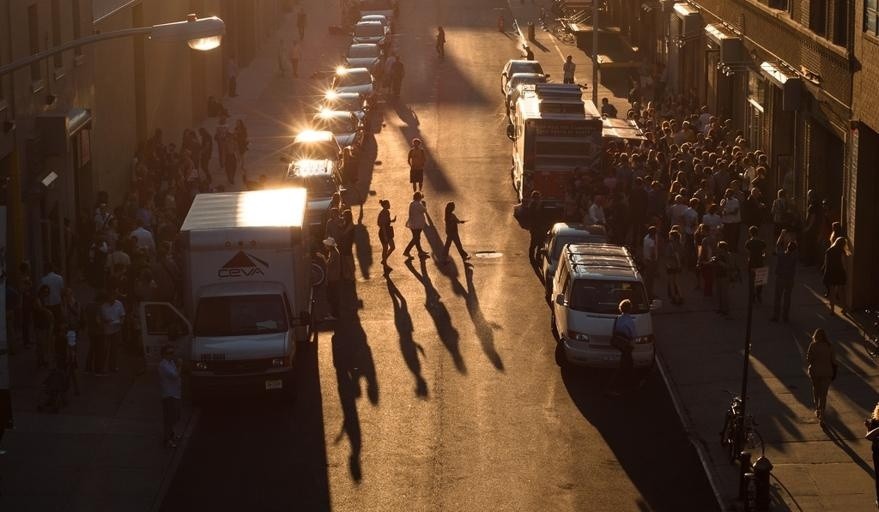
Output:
<box><xmin>276</xmin><ymin>8</ymin><xmax>308</xmax><ymax>78</ymax></box>
<box><xmin>615</xmin><ymin>299</ymin><xmax>644</xmax><ymax>395</ymax></box>
<box><xmin>527</xmin><ymin>191</ymin><xmax>546</xmax><ymax>263</ymax></box>
<box><xmin>15</xmin><ymin>96</ymin><xmax>270</xmax><ymax>451</ymax></box>
<box><xmin>226</xmin><ymin>55</ymin><xmax>240</xmax><ymax>98</ymax></box>
<box><xmin>806</xmin><ymin>327</ymin><xmax>835</xmax><ymax>428</ymax></box>
<box><xmin>525</xmin><ymin>47</ymin><xmax>534</xmax><ymax>60</ymax></box>
<box><xmin>316</xmin><ymin>193</ymin><xmax>357</xmax><ymax>320</ymax></box>
<box><xmin>563</xmin><ymin>55</ymin><xmax>576</xmax><ymax>84</ymax></box>
<box><xmin>435</xmin><ymin>28</ymin><xmax>446</xmax><ymax>58</ymax></box>
<box><xmin>563</xmin><ymin>87</ymin><xmax>850</xmax><ymax>326</ymax></box>
<box><xmin>377</xmin><ymin>192</ymin><xmax>472</xmax><ymax>265</ymax></box>
<box><xmin>408</xmin><ymin>138</ymin><xmax>426</xmax><ymax>192</ymax></box>
<box><xmin>385</xmin><ymin>52</ymin><xmax>406</xmax><ymax>96</ymax></box>
<box><xmin>866</xmin><ymin>404</ymin><xmax>879</xmax><ymax>505</ymax></box>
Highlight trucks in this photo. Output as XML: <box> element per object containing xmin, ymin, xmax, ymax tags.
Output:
<box><xmin>137</xmin><ymin>183</ymin><xmax>321</xmax><ymax>407</ymax></box>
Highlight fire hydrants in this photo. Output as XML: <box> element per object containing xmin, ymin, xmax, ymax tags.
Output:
<box><xmin>745</xmin><ymin>455</ymin><xmax>775</xmax><ymax>511</ymax></box>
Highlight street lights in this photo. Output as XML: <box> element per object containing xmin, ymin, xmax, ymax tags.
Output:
<box><xmin>1</xmin><ymin>12</ymin><xmax>228</xmax><ymax>76</ymax></box>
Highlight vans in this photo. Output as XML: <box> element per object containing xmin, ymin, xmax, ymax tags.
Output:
<box><xmin>549</xmin><ymin>238</ymin><xmax>656</xmax><ymax>378</ymax></box>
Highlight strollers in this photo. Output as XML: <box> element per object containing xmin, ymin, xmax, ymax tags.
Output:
<box><xmin>34</xmin><ymin>363</ymin><xmax>74</xmax><ymax>417</ymax></box>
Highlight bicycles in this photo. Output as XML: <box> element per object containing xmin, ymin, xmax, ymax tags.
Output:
<box><xmin>861</xmin><ymin>303</ymin><xmax>878</xmax><ymax>358</ymax></box>
<box><xmin>715</xmin><ymin>388</ymin><xmax>750</xmax><ymax>469</ymax></box>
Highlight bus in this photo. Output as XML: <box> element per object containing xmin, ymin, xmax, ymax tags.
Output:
<box><xmin>507</xmin><ymin>81</ymin><xmax>649</xmax><ymax>214</ymax></box>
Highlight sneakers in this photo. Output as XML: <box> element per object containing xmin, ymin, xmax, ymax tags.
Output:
<box><xmin>463</xmin><ymin>256</ymin><xmax>471</xmax><ymax>261</ymax></box>
<box><xmin>418</xmin><ymin>251</ymin><xmax>429</xmax><ymax>257</ymax></box>
<box><xmin>403</xmin><ymin>252</ymin><xmax>414</xmax><ymax>258</ymax></box>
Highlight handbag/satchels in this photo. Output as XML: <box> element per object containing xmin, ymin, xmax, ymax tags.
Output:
<box><xmin>386</xmin><ymin>224</ymin><xmax>394</xmax><ymax>238</ymax></box>
<box><xmin>611</xmin><ymin>335</ymin><xmax>634</xmax><ymax>353</ymax></box>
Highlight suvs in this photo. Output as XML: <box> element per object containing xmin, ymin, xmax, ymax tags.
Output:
<box><xmin>280</xmin><ymin>158</ymin><xmax>346</xmax><ymax>236</ymax></box>
<box><xmin>288</xmin><ymin>128</ymin><xmax>347</xmax><ymax>181</ymax></box>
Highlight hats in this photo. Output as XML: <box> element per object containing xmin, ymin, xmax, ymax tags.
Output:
<box><xmin>322</xmin><ymin>237</ymin><xmax>337</xmax><ymax>247</ymax></box>
<box><xmin>413</xmin><ymin>191</ymin><xmax>424</xmax><ymax>199</ymax></box>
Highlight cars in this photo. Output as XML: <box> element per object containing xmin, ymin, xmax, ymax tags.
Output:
<box><xmin>538</xmin><ymin>219</ymin><xmax>615</xmax><ymax>302</ymax></box>
<box><xmin>306</xmin><ymin>111</ymin><xmax>366</xmax><ymax>159</ymax></box>
<box><xmin>500</xmin><ymin>58</ymin><xmax>551</xmax><ymax>99</ymax></box>
<box><xmin>322</xmin><ymin>0</ymin><xmax>398</xmax><ymax>135</ymax></box>
<box><xmin>504</xmin><ymin>72</ymin><xmax>548</xmax><ymax>117</ymax></box>
